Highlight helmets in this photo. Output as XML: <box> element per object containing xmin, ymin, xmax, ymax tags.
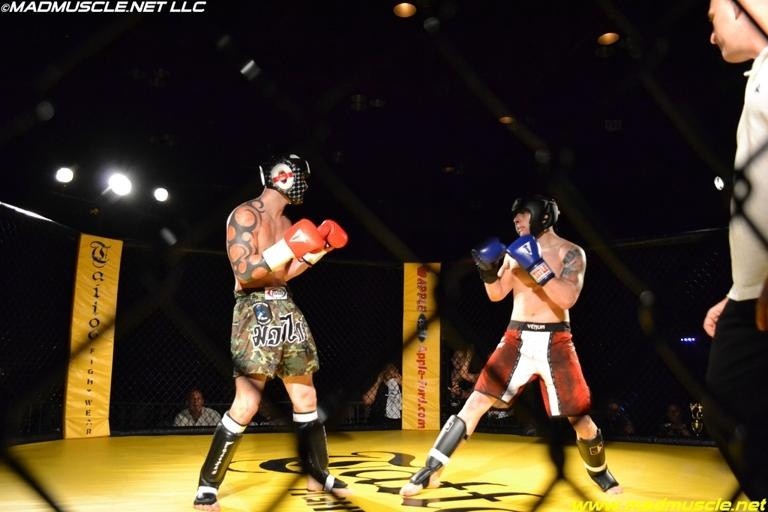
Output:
<box><xmin>512</xmin><ymin>194</ymin><xmax>560</xmax><ymax>236</ymax></box>
<box><xmin>260</xmin><ymin>151</ymin><xmax>311</xmax><ymax>205</ymax></box>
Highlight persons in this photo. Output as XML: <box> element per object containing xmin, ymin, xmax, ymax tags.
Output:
<box><xmin>400</xmin><ymin>189</ymin><xmax>625</xmax><ymax>498</ymax></box>
<box><xmin>700</xmin><ymin>0</ymin><xmax>768</xmax><ymax>510</ymax></box>
<box><xmin>192</xmin><ymin>148</ymin><xmax>355</xmax><ymax>512</ymax></box>
<box><xmin>173</xmin><ymin>342</ymin><xmax>693</xmax><ymax>439</ymax></box>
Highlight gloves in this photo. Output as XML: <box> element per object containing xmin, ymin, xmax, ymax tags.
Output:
<box><xmin>507</xmin><ymin>234</ymin><xmax>554</xmax><ymax>286</ymax></box>
<box><xmin>471</xmin><ymin>236</ymin><xmax>507</xmax><ymax>284</ymax></box>
<box><xmin>261</xmin><ymin>219</ymin><xmax>348</xmax><ymax>271</ymax></box>
